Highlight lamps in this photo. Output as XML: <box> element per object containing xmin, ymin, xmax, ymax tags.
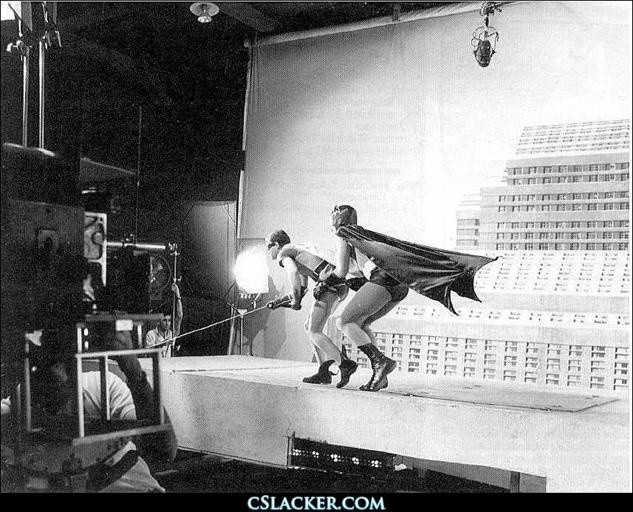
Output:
<box><xmin>470</xmin><ymin>1</ymin><xmax>511</xmax><ymax>68</ymax></box>
<box><xmin>188</xmin><ymin>2</ymin><xmax>220</xmax><ymax>24</ymax></box>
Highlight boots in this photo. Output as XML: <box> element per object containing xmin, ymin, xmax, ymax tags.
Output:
<box><xmin>336</xmin><ymin>344</ymin><xmax>358</xmax><ymax>388</ymax></box>
<box><xmin>303</xmin><ymin>359</ymin><xmax>339</xmax><ymax>384</ymax></box>
<box><xmin>357</xmin><ymin>343</ymin><xmax>397</xmax><ymax>392</ymax></box>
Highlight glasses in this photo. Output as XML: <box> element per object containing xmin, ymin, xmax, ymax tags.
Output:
<box><xmin>267</xmin><ymin>243</ymin><xmax>275</xmax><ymax>250</ymax></box>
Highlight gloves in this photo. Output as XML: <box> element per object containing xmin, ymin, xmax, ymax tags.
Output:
<box><xmin>313</xmin><ymin>273</ymin><xmax>346</xmax><ymax>300</ymax></box>
<box><xmin>288</xmin><ymin>286</ymin><xmax>308</xmax><ymax>310</ymax></box>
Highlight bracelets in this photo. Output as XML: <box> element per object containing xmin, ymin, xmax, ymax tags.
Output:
<box><xmin>175</xmin><ymin>297</ymin><xmax>182</xmax><ymax>299</ymax></box>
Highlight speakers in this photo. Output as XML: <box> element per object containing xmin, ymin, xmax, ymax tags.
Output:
<box><xmin>0</xmin><ymin>199</ymin><xmax>86</xmax><ymax>328</ymax></box>
<box><xmin>150</xmin><ymin>248</ymin><xmax>183</xmax><ymax>300</ymax></box>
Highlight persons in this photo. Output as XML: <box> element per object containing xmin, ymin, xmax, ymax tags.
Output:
<box><xmin>145</xmin><ymin>282</ymin><xmax>183</xmax><ymax>358</ymax></box>
<box><xmin>1</xmin><ymin>358</ymin><xmax>165</xmax><ymax>493</ymax></box>
<box><xmin>313</xmin><ymin>205</ymin><xmax>498</xmax><ymax>392</ymax></box>
<box><xmin>266</xmin><ymin>229</ymin><xmax>358</xmax><ymax>388</ymax></box>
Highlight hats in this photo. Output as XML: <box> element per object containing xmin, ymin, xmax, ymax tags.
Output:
<box><xmin>265</xmin><ymin>229</ymin><xmax>290</xmax><ymax>249</ymax></box>
<box><xmin>329</xmin><ymin>205</ymin><xmax>357</xmax><ymax>231</ymax></box>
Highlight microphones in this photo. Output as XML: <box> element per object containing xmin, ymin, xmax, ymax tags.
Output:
<box><xmin>474</xmin><ymin>39</ymin><xmax>496</xmax><ymax>66</ymax></box>
